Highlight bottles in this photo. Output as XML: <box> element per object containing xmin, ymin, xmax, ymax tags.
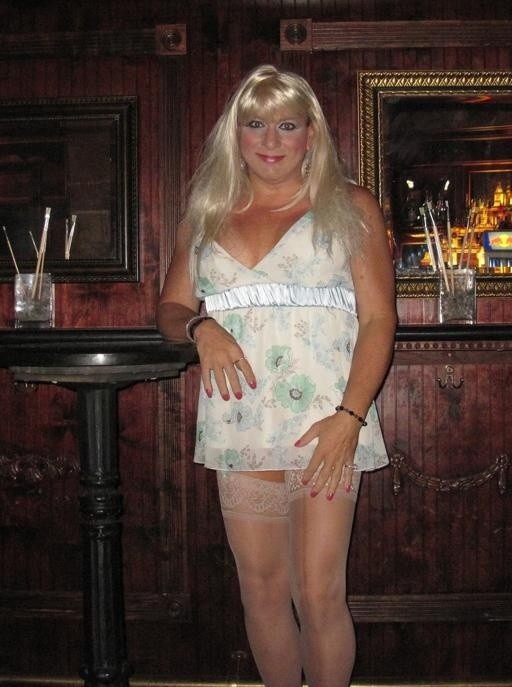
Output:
<box><xmin>492</xmin><ymin>182</ymin><xmax>512</xmax><ymax>207</ymax></box>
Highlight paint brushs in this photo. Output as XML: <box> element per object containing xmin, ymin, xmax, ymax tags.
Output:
<box><xmin>2</xmin><ymin>206</ymin><xmax>51</xmax><ymax>300</ymax></box>
<box><xmin>419</xmin><ymin>200</ymin><xmax>477</xmax><ymax>297</ymax></box>
<box><xmin>64</xmin><ymin>214</ymin><xmax>77</xmax><ymax>260</ymax></box>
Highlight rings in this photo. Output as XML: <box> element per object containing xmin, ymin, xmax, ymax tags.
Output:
<box><xmin>345</xmin><ymin>463</ymin><xmax>357</xmax><ymax>469</ymax></box>
<box><xmin>235</xmin><ymin>357</ymin><xmax>245</xmax><ymax>363</ymax></box>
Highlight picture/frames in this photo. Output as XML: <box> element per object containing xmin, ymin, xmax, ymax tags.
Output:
<box><xmin>0</xmin><ymin>94</ymin><xmax>140</xmax><ymax>283</ymax></box>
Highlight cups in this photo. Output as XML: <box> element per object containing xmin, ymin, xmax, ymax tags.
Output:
<box><xmin>14</xmin><ymin>274</ymin><xmax>52</xmax><ymax>329</ymax></box>
<box><xmin>437</xmin><ymin>267</ymin><xmax>477</xmax><ymax>324</ymax></box>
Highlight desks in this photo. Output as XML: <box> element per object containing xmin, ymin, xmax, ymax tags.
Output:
<box><xmin>0</xmin><ymin>342</ymin><xmax>199</xmax><ymax>686</ymax></box>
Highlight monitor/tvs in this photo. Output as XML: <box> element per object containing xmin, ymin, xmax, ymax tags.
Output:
<box><xmin>400</xmin><ymin>242</ymin><xmax>438</xmax><ymax>270</ymax></box>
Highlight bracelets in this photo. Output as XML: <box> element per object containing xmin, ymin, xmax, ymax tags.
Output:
<box><xmin>185</xmin><ymin>314</ymin><xmax>208</xmax><ymax>344</ymax></box>
<box><xmin>334</xmin><ymin>406</ymin><xmax>368</xmax><ymax>429</ymax></box>
<box><xmin>189</xmin><ymin>316</ymin><xmax>215</xmax><ymax>339</ymax></box>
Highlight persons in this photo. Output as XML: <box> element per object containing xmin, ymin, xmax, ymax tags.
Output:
<box><xmin>155</xmin><ymin>63</ymin><xmax>400</xmax><ymax>686</ymax></box>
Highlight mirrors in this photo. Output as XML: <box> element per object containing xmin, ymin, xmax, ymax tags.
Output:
<box><xmin>355</xmin><ymin>66</ymin><xmax>511</xmax><ymax>298</ymax></box>
